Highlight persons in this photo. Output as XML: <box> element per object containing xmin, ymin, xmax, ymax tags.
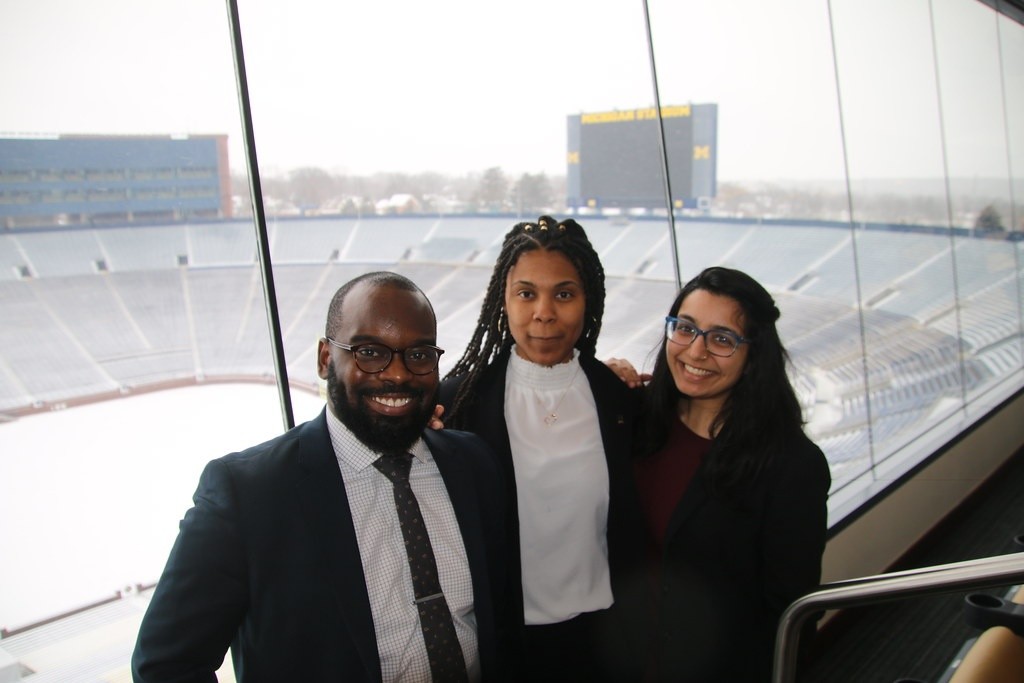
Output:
<box><xmin>130</xmin><ymin>270</ymin><xmax>511</xmax><ymax>682</ymax></box>
<box><xmin>426</xmin><ymin>214</ymin><xmax>666</xmax><ymax>683</ymax></box>
<box><xmin>605</xmin><ymin>266</ymin><xmax>831</xmax><ymax>683</ymax></box>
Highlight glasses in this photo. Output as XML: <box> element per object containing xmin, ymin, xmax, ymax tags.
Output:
<box><xmin>665</xmin><ymin>316</ymin><xmax>752</xmax><ymax>357</ymax></box>
<box><xmin>327</xmin><ymin>337</ymin><xmax>444</xmax><ymax>375</ymax></box>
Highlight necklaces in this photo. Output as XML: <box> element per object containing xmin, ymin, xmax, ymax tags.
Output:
<box><xmin>513</xmin><ymin>364</ymin><xmax>580</xmax><ymax>426</ymax></box>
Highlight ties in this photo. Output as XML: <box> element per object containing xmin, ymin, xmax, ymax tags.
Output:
<box><xmin>372</xmin><ymin>453</ymin><xmax>468</xmax><ymax>683</ymax></box>
